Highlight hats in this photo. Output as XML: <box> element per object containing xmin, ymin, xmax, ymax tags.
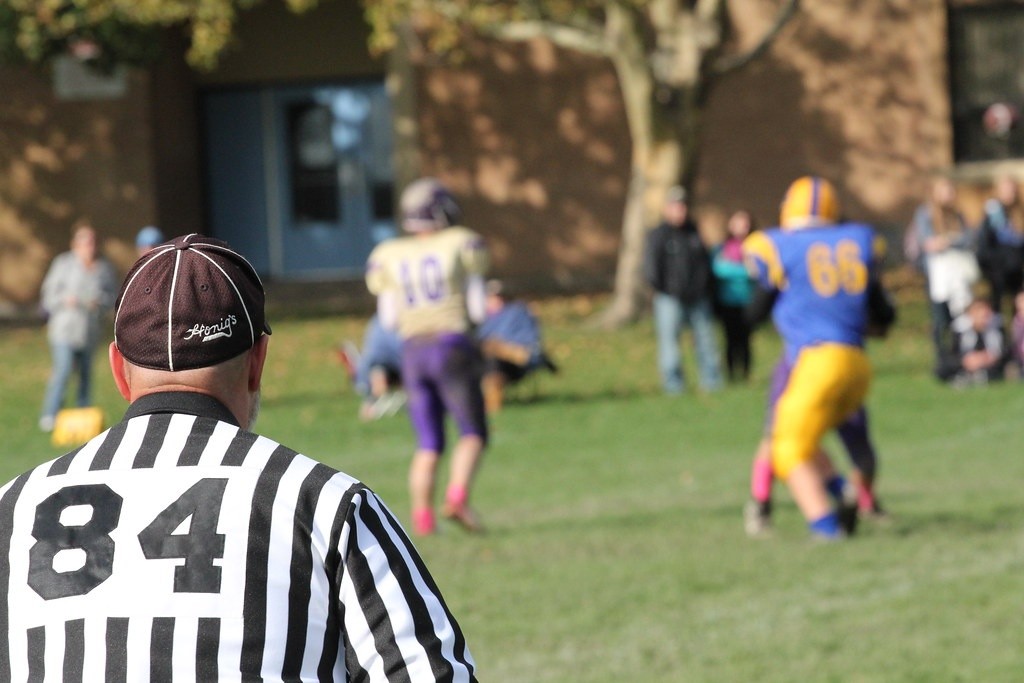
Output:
<box><xmin>114</xmin><ymin>232</ymin><xmax>272</xmax><ymax>371</ymax></box>
<box><xmin>136</xmin><ymin>226</ymin><xmax>162</xmax><ymax>246</ymax></box>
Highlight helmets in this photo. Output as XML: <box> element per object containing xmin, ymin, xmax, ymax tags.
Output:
<box><xmin>398</xmin><ymin>176</ymin><xmax>454</xmax><ymax>230</ymax></box>
<box><xmin>780</xmin><ymin>175</ymin><xmax>838</xmax><ymax>229</ymax></box>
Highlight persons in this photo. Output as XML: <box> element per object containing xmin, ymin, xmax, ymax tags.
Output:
<box><xmin>646</xmin><ymin>177</ymin><xmax>900</xmax><ymax>539</ymax></box>
<box><xmin>0</xmin><ymin>233</ymin><xmax>475</xmax><ymax>683</ymax></box>
<box><xmin>357</xmin><ymin>180</ymin><xmax>542</xmax><ymax>536</ymax></box>
<box><xmin>905</xmin><ymin>178</ymin><xmax>1024</xmax><ymax>384</ymax></box>
<box><xmin>41</xmin><ymin>222</ymin><xmax>160</xmax><ymax>430</ymax></box>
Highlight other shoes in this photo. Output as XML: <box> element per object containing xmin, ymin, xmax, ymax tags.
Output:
<box><xmin>849</xmin><ymin>470</ymin><xmax>876</xmax><ymax>520</ymax></box>
<box><xmin>743</xmin><ymin>499</ymin><xmax>771</xmax><ymax>537</ymax></box>
<box><xmin>442</xmin><ymin>507</ymin><xmax>481</xmax><ymax>531</ymax></box>
